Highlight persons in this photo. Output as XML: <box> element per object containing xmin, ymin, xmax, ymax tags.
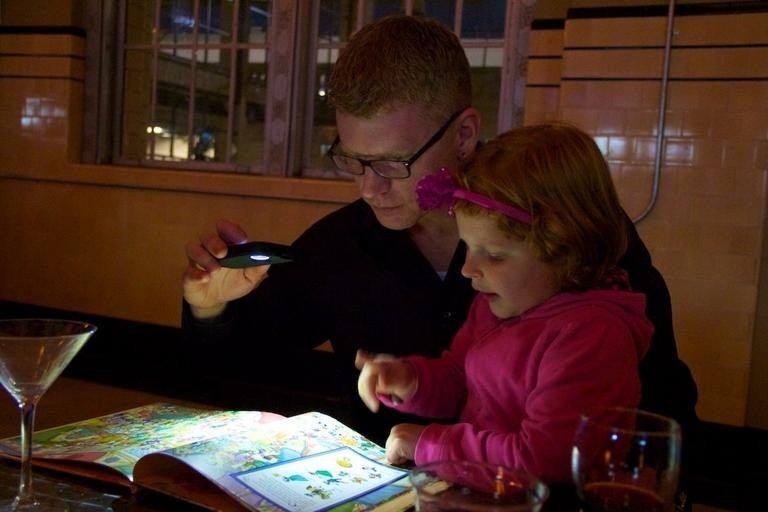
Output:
<box><xmin>347</xmin><ymin>120</ymin><xmax>656</xmax><ymax>502</ymax></box>
<box><xmin>179</xmin><ymin>13</ymin><xmax>716</xmax><ymax>512</ymax></box>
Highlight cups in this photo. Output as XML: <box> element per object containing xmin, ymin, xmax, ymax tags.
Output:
<box><xmin>572</xmin><ymin>407</ymin><xmax>681</xmax><ymax>511</ymax></box>
<box><xmin>410</xmin><ymin>460</ymin><xmax>551</xmax><ymax>512</ymax></box>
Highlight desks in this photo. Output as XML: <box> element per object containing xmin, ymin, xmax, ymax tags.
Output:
<box><xmin>0</xmin><ymin>361</ymin><xmax>579</xmax><ymax>509</ymax></box>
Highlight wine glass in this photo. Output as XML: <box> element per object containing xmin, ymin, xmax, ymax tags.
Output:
<box><xmin>0</xmin><ymin>318</ymin><xmax>98</xmax><ymax>512</ymax></box>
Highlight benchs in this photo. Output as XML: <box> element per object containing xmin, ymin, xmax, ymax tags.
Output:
<box><xmin>0</xmin><ymin>297</ymin><xmax>764</xmax><ymax>509</ymax></box>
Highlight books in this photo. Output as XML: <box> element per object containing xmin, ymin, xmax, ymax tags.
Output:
<box><xmin>0</xmin><ymin>401</ymin><xmax>456</xmax><ymax>511</ymax></box>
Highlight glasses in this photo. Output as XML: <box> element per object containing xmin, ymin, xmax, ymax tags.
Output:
<box><xmin>325</xmin><ymin>105</ymin><xmax>467</xmax><ymax>181</ymax></box>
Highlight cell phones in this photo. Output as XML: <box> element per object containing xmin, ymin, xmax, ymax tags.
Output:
<box><xmin>220</xmin><ymin>241</ymin><xmax>296</xmax><ymax>267</ymax></box>
